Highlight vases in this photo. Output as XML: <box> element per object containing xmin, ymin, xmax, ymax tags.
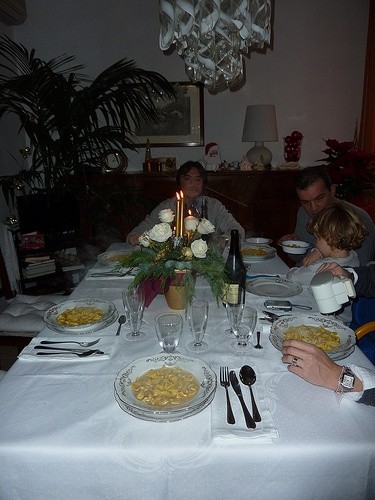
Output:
<box><xmin>164</xmin><ymin>269</ymin><xmax>197</xmax><ymax>311</ymax></box>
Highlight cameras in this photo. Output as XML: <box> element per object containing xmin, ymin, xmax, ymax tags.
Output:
<box><xmin>263</xmin><ymin>300</ymin><xmax>292</xmax><ymax>312</ymax></box>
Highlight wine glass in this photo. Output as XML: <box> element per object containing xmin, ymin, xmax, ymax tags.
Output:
<box><xmin>154</xmin><ymin>312</ymin><xmax>183</xmax><ymax>353</ymax></box>
<box><xmin>122</xmin><ymin>289</ymin><xmax>147</xmax><ymax>342</ymax></box>
<box><xmin>224</xmin><ymin>286</ymin><xmax>244</xmax><ymax>338</ymax></box>
<box><xmin>230</xmin><ymin>304</ymin><xmax>257</xmax><ymax>353</ymax></box>
<box><xmin>185</xmin><ymin>299</ymin><xmax>209</xmax><ymax>353</ymax></box>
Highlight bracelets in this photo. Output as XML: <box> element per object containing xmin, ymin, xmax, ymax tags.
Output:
<box><xmin>334</xmin><ymin>365</ymin><xmax>345</xmax><ymax>392</ymax></box>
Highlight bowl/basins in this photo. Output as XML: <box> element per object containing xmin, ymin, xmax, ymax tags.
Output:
<box><xmin>279</xmin><ymin>240</ymin><xmax>312</xmax><ymax>254</ymax></box>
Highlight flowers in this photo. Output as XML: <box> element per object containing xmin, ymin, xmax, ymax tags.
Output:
<box><xmin>97</xmin><ymin>208</ymin><xmax>232</xmax><ymax>308</ymax></box>
<box><xmin>314</xmin><ymin>136</ymin><xmax>375</xmax><ymax>194</ymax></box>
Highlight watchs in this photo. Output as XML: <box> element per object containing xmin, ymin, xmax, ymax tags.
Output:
<box><xmin>340</xmin><ymin>369</ymin><xmax>355</xmax><ymax>392</ymax></box>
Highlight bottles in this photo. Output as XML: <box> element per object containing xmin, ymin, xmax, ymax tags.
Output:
<box><xmin>222</xmin><ymin>229</ymin><xmax>246</xmax><ymax>308</ymax></box>
<box><xmin>201</xmin><ymin>199</ymin><xmax>210</xmax><ymax>245</ymax></box>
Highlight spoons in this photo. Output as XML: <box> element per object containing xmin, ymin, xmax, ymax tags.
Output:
<box><xmin>262</xmin><ymin>311</ymin><xmax>292</xmax><ymax>317</ymax></box>
<box><xmin>116</xmin><ymin>315</ymin><xmax>127</xmax><ymax>336</ymax></box>
<box><xmin>239</xmin><ymin>365</ymin><xmax>262</xmax><ymax>421</ymax></box>
<box><xmin>255</xmin><ymin>331</ymin><xmax>263</xmax><ymax>349</ymax></box>
<box><xmin>36</xmin><ymin>350</ymin><xmax>98</xmax><ymax>358</ymax></box>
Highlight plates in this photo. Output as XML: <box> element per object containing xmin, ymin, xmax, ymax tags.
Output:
<box><xmin>42</xmin><ymin>298</ymin><xmax>119</xmax><ymax>335</ymax></box>
<box><xmin>241</xmin><ymin>237</ymin><xmax>277</xmax><ymax>261</ymax></box>
<box><xmin>269</xmin><ymin>314</ymin><xmax>356</xmax><ymax>361</ymax></box>
<box><xmin>245</xmin><ymin>277</ymin><xmax>304</xmax><ymax>297</ymax></box>
<box><xmin>113</xmin><ymin>352</ymin><xmax>217</xmax><ymax>423</ymax></box>
<box><xmin>98</xmin><ymin>250</ymin><xmax>132</xmax><ymax>267</ymax></box>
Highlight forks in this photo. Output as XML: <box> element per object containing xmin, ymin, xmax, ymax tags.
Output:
<box><xmin>40</xmin><ymin>338</ymin><xmax>101</xmax><ymax>347</ymax></box>
<box><xmin>219</xmin><ymin>366</ymin><xmax>235</xmax><ymax>425</ymax></box>
<box><xmin>91</xmin><ymin>268</ymin><xmax>134</xmax><ymax>274</ymax></box>
<box><xmin>265</xmin><ymin>317</ymin><xmax>274</xmax><ymax>323</ymax></box>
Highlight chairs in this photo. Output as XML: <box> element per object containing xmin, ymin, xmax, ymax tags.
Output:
<box><xmin>0</xmin><ymin>246</ymin><xmax>70</xmax><ymax>346</ymax></box>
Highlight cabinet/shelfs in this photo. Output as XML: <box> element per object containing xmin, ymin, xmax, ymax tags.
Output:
<box><xmin>75</xmin><ymin>167</ymin><xmax>303</xmax><ymax>265</ymax></box>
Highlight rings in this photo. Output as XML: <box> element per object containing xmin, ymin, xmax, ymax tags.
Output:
<box><xmin>293</xmin><ymin>357</ymin><xmax>298</xmax><ymax>366</ymax></box>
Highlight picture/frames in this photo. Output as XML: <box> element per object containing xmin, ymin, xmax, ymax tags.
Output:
<box><xmin>120</xmin><ymin>81</ymin><xmax>206</xmax><ymax>149</ymax></box>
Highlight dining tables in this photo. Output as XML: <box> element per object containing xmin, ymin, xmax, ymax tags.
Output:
<box><xmin>0</xmin><ymin>243</ymin><xmax>375</xmax><ymax>500</ymax></box>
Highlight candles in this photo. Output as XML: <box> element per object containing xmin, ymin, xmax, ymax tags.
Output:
<box><xmin>176</xmin><ymin>192</ymin><xmax>180</xmax><ymax>237</ymax></box>
<box><xmin>180</xmin><ymin>190</ymin><xmax>184</xmax><ymax>237</ymax></box>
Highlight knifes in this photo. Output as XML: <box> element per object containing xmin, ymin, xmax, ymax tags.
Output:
<box><xmin>33</xmin><ymin>345</ymin><xmax>104</xmax><ymax>354</ymax></box>
<box><xmin>230</xmin><ymin>370</ymin><xmax>257</xmax><ymax>430</ymax></box>
<box><xmin>260</xmin><ymin>317</ymin><xmax>278</xmax><ymax>320</ymax></box>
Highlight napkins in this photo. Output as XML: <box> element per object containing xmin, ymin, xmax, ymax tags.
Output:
<box><xmin>210</xmin><ymin>365</ymin><xmax>281</xmax><ymax>443</ymax></box>
<box><xmin>17</xmin><ymin>335</ymin><xmax>111</xmax><ymax>362</ymax></box>
<box><xmin>84</xmin><ymin>269</ymin><xmax>135</xmax><ymax>280</ymax></box>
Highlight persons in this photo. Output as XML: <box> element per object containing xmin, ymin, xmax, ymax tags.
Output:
<box><xmin>285</xmin><ymin>200</ymin><xmax>365</xmax><ymax>328</ymax></box>
<box><xmin>281</xmin><ymin>261</ymin><xmax>375</xmax><ymax>407</ymax></box>
<box><xmin>276</xmin><ymin>170</ymin><xmax>375</xmax><ymax>266</ymax></box>
<box><xmin>127</xmin><ymin>161</ymin><xmax>246</xmax><ymax>246</ymax></box>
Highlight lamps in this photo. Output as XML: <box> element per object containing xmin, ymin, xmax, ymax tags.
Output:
<box><xmin>241</xmin><ymin>103</ymin><xmax>278</xmax><ymax>167</ymax></box>
<box><xmin>157</xmin><ymin>0</ymin><xmax>272</xmax><ymax>93</ymax></box>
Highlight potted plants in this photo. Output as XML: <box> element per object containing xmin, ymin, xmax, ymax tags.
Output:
<box><xmin>1</xmin><ymin>32</ymin><xmax>178</xmax><ymax>235</ymax></box>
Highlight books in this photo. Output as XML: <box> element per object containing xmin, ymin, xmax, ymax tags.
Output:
<box><xmin>57</xmin><ymin>256</ymin><xmax>85</xmax><ymax>272</ymax></box>
<box><xmin>22</xmin><ymin>254</ymin><xmax>56</xmax><ymax>278</ymax></box>
